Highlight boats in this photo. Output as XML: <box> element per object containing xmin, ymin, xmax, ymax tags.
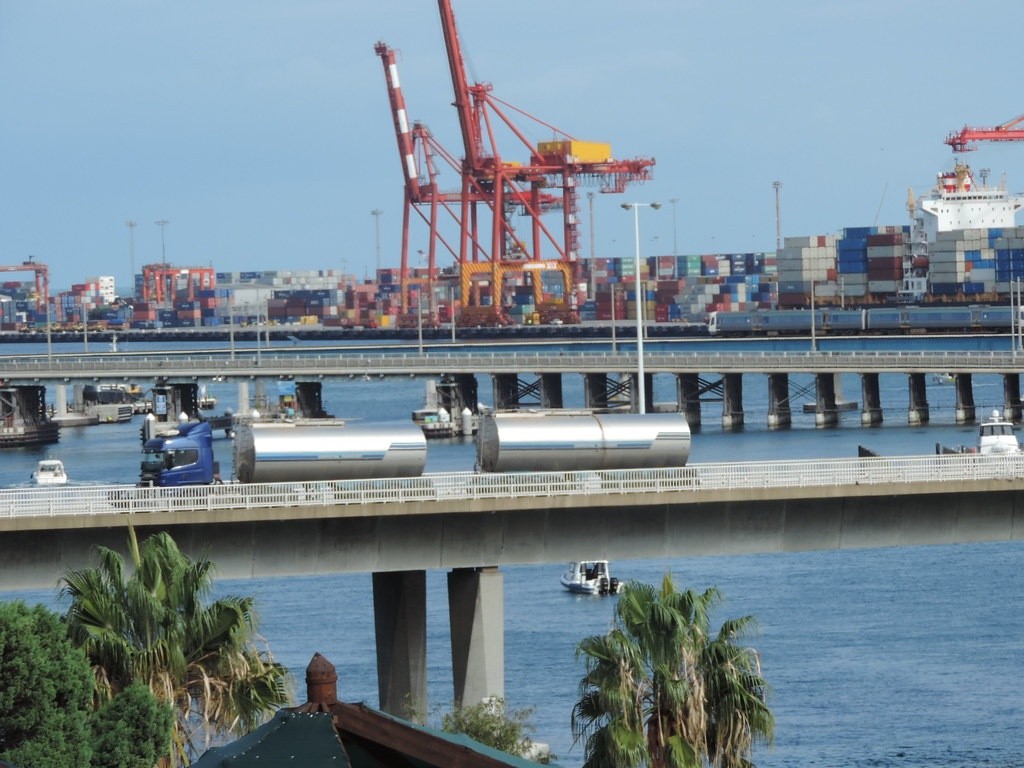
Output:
<box><xmin>556</xmin><ymin>558</ymin><xmax>612</xmax><ymax>596</ymax></box>
<box><xmin>977</xmin><ymin>407</ymin><xmax>1020</xmax><ymax>456</ymax></box>
<box><xmin>30</xmin><ymin>458</ymin><xmax>69</xmax><ymax>486</ymax></box>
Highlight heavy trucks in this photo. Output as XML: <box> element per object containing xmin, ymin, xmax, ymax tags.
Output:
<box><xmin>138</xmin><ymin>414</ymin><xmax>427</xmax><ymax>480</ymax></box>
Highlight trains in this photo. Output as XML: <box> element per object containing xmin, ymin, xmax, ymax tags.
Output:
<box><xmin>708</xmin><ymin>302</ymin><xmax>1024</xmax><ymax>341</ymax></box>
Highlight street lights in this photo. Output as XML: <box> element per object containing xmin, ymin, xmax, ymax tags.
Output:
<box><xmin>586</xmin><ymin>190</ymin><xmax>596</xmax><ymax>300</ymax></box>
<box><xmin>370</xmin><ymin>208</ymin><xmax>383</xmax><ymax>275</ymax></box>
<box><xmin>156</xmin><ymin>218</ymin><xmax>168</xmax><ymax>274</ymax></box>
<box><xmin>773</xmin><ymin>180</ymin><xmax>782</xmax><ymax>251</ymax></box>
<box><xmin>623</xmin><ymin>200</ymin><xmax>663</xmax><ymax>418</ymax></box>
<box><xmin>126</xmin><ymin>218</ymin><xmax>137</xmax><ymax>287</ymax></box>
